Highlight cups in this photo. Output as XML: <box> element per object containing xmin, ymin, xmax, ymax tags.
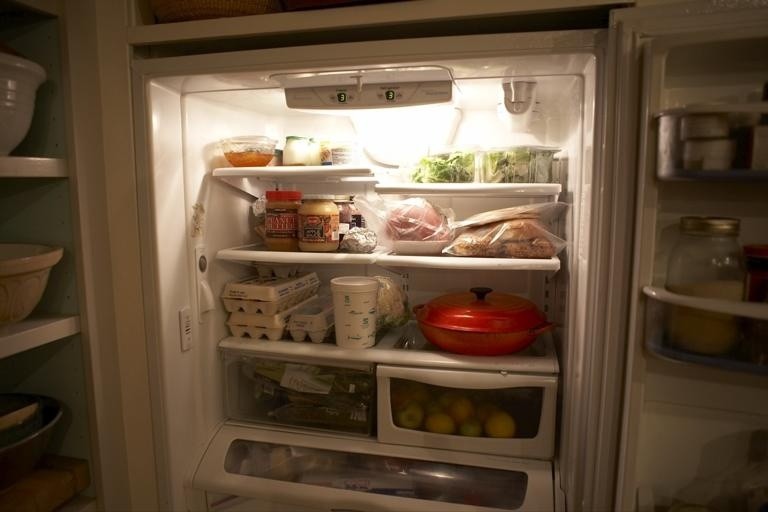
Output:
<box><xmin>330</xmin><ymin>276</ymin><xmax>379</xmax><ymax>351</ymax></box>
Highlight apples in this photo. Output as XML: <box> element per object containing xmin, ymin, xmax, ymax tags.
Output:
<box><xmin>391</xmin><ymin>380</ymin><xmax>516</xmax><ymax>438</ymax></box>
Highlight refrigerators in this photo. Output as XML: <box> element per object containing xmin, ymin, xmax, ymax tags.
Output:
<box><xmin>123</xmin><ymin>1</ymin><xmax>767</xmax><ymax>512</ymax></box>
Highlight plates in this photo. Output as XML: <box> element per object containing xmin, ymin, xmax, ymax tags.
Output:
<box><xmin>392</xmin><ymin>238</ymin><xmax>448</xmax><ymax>255</ymax></box>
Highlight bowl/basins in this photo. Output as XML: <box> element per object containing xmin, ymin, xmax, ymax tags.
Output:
<box><xmin>0</xmin><ymin>53</ymin><xmax>48</xmax><ymax>153</ymax></box>
<box><xmin>0</xmin><ymin>243</ymin><xmax>65</xmax><ymax>322</ymax></box>
<box><xmin>412</xmin><ymin>286</ymin><xmax>557</xmax><ymax>358</ymax></box>
<box><xmin>0</xmin><ymin>394</ymin><xmax>65</xmax><ymax>487</ymax></box>
<box><xmin>221</xmin><ymin>135</ymin><xmax>278</xmax><ymax>167</ymax></box>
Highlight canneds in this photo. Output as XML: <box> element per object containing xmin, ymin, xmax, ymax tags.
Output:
<box><xmin>265</xmin><ymin>191</ymin><xmax>366</xmax><ymax>252</ymax></box>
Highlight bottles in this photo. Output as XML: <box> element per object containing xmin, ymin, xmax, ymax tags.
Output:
<box><xmin>282</xmin><ymin>135</ymin><xmax>316</xmax><ymax>165</ymax></box>
<box><xmin>665</xmin><ymin>214</ymin><xmax>742</xmax><ymax>363</ymax></box>
